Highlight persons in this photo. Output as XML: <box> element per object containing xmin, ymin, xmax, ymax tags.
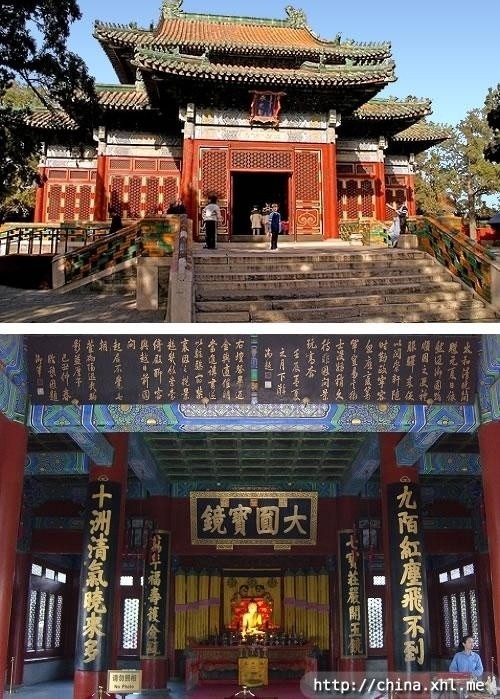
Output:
<box><xmin>108</xmin><ymin>207</ymin><xmax>123</xmax><ymax>234</ymax></box>
<box><xmin>250</xmin><ymin>202</ymin><xmax>282</xmax><ymax>251</ymax></box>
<box><xmin>449</xmin><ymin>636</ymin><xmax>483</xmax><ymax>679</ymax></box>
<box><xmin>241</xmin><ymin>603</ymin><xmax>265</xmax><ymax>641</ymax></box>
<box><xmin>166</xmin><ymin>194</ymin><xmax>223</xmax><ymax>250</ymax></box>
<box><xmin>386</xmin><ymin>201</ymin><xmax>408</xmax><ymax>248</ymax></box>
